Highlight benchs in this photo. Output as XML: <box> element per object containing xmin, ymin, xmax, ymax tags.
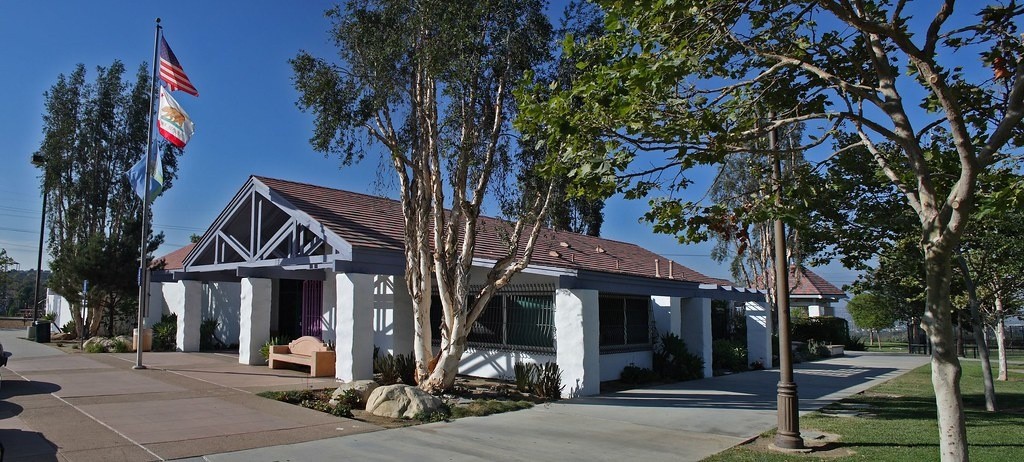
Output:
<box><xmin>267</xmin><ymin>334</ymin><xmax>336</xmax><ymax>379</ymax></box>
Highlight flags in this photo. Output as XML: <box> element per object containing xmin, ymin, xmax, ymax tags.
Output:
<box><xmin>158</xmin><ymin>27</ymin><xmax>199</xmax><ymax>97</ymax></box>
<box><xmin>157</xmin><ymin>83</ymin><xmax>195</xmax><ymax>149</ymax></box>
<box><xmin>125</xmin><ymin>136</ymin><xmax>164</xmax><ymax>205</ymax></box>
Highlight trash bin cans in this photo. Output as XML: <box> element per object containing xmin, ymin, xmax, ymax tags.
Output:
<box><xmin>35</xmin><ymin>320</ymin><xmax>50</xmax><ymax>343</ymax></box>
<box><xmin>133</xmin><ymin>329</ymin><xmax>152</xmax><ymax>352</ymax></box>
